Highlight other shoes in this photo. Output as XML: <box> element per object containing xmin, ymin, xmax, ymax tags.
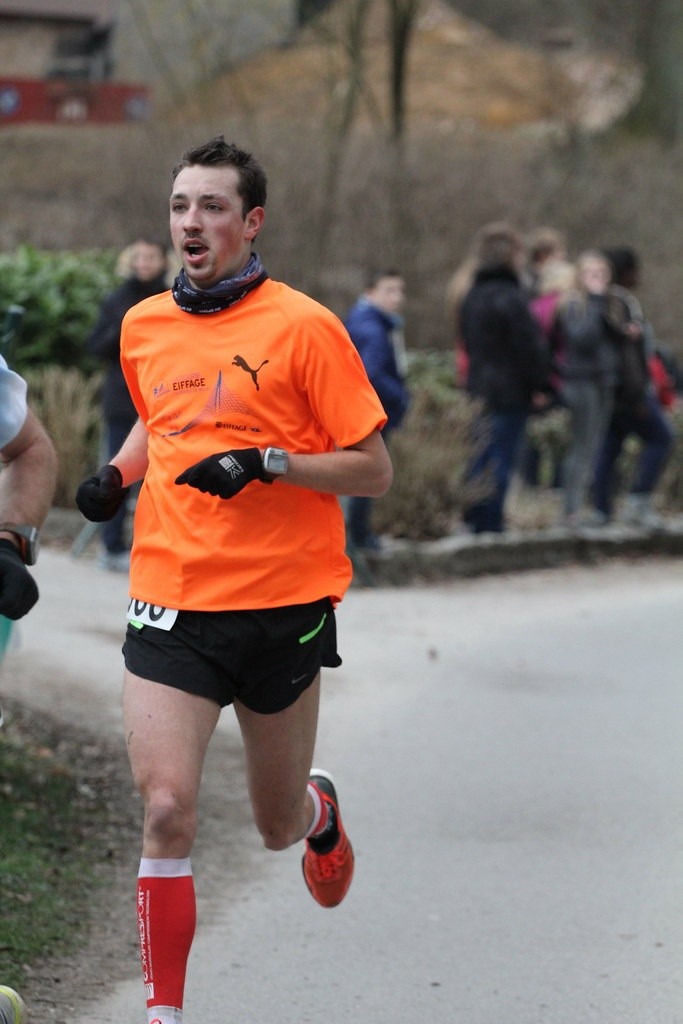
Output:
<box><xmin>0</xmin><ymin>986</ymin><xmax>25</xmax><ymax>1024</ymax></box>
<box><xmin>98</xmin><ymin>551</ymin><xmax>131</xmax><ymax>572</ymax></box>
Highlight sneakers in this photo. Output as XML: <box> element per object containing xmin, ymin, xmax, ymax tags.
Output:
<box><xmin>301</xmin><ymin>768</ymin><xmax>353</xmax><ymax>909</ymax></box>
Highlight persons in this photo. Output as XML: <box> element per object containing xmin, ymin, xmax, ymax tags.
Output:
<box><xmin>445</xmin><ymin>220</ymin><xmax>683</xmax><ymax>533</ymax></box>
<box><xmin>73</xmin><ymin>135</ymin><xmax>395</xmax><ymax>1024</ymax></box>
<box><xmin>345</xmin><ymin>265</ymin><xmax>413</xmax><ymax>587</ymax></box>
<box><xmin>84</xmin><ymin>235</ymin><xmax>170</xmax><ymax>568</ymax></box>
<box><xmin>0</xmin><ymin>350</ymin><xmax>57</xmax><ymax>658</ymax></box>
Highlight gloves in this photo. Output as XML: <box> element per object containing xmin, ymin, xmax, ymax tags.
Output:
<box><xmin>0</xmin><ymin>538</ymin><xmax>39</xmax><ymax>620</ymax></box>
<box><xmin>76</xmin><ymin>464</ymin><xmax>128</xmax><ymax>523</ymax></box>
<box><xmin>175</xmin><ymin>449</ymin><xmax>262</xmax><ymax>499</ymax></box>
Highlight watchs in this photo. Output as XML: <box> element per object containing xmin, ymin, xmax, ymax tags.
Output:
<box><xmin>263</xmin><ymin>447</ymin><xmax>289</xmax><ymax>475</ymax></box>
<box><xmin>0</xmin><ymin>521</ymin><xmax>40</xmax><ymax>566</ymax></box>
